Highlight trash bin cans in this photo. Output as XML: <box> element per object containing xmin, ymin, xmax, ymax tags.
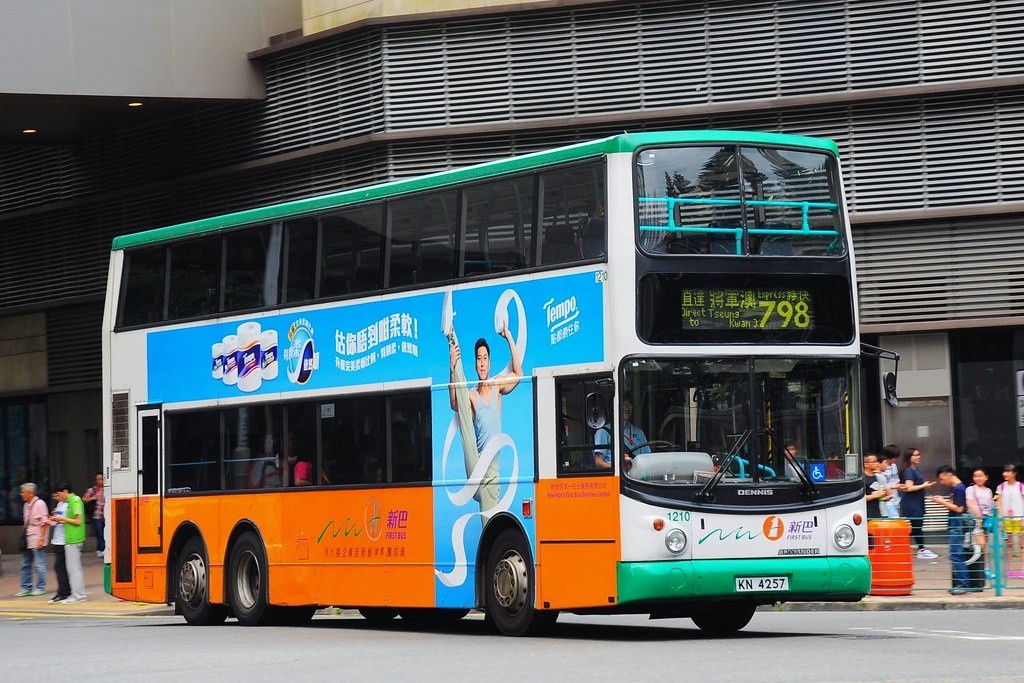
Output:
<box><xmin>867</xmin><ymin>520</ymin><xmax>915</xmax><ymax>595</ymax></box>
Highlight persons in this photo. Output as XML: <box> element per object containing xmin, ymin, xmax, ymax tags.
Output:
<box><xmin>14</xmin><ymin>473</ymin><xmax>104</xmax><ymax>603</ymax></box>
<box><xmin>784</xmin><ymin>438</ymin><xmax>1024</xmax><ymax>596</ymax></box>
<box><xmin>594</xmin><ymin>391</ymin><xmax>651</xmax><ymax>470</ymax></box>
<box><xmin>442</xmin><ymin>290</ymin><xmax>524</xmax><ymax>527</ymax></box>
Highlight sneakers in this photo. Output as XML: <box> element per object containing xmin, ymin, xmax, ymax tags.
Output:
<box><xmin>440</xmin><ymin>289</ymin><xmax>456</xmax><ymax>337</ymax></box>
<box><xmin>83</xmin><ymin>490</ymin><xmax>97</xmax><ymax>520</ymax></box>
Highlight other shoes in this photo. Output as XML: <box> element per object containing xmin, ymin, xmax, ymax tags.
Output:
<box><xmin>1007</xmin><ymin>571</ymin><xmax>1017</xmax><ymax>578</ymax></box>
<box><xmin>984</xmin><ymin>569</ymin><xmax>997</xmax><ymax>578</ymax></box>
<box><xmin>30</xmin><ymin>587</ymin><xmax>46</xmax><ymax>596</ymax></box>
<box><xmin>916</xmin><ymin>549</ymin><xmax>939</xmax><ymax>559</ymax></box>
<box><xmin>48</xmin><ymin>595</ymin><xmax>65</xmax><ymax>603</ymax></box>
<box><xmin>14</xmin><ymin>589</ymin><xmax>32</xmax><ymax>598</ymax></box>
<box><xmin>949</xmin><ymin>587</ymin><xmax>966</xmax><ymax>593</ymax></box>
<box><xmin>1018</xmin><ymin>572</ymin><xmax>1024</xmax><ymax>579</ymax></box>
<box><xmin>62</xmin><ymin>595</ymin><xmax>87</xmax><ymax>603</ymax></box>
<box><xmin>97</xmin><ymin>550</ymin><xmax>104</xmax><ymax>557</ymax></box>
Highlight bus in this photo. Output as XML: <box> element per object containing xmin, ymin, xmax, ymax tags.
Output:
<box><xmin>101</xmin><ymin>128</ymin><xmax>900</xmax><ymax>632</ymax></box>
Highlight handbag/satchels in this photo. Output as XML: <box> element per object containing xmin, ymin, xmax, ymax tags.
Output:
<box><xmin>19</xmin><ymin>530</ymin><xmax>27</xmax><ymax>551</ymax></box>
<box><xmin>961</xmin><ymin>513</ymin><xmax>976</xmax><ymax>534</ymax></box>
<box><xmin>982</xmin><ymin>514</ymin><xmax>993</xmax><ymax>533</ymax></box>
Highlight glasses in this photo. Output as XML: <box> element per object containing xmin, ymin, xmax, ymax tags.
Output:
<box><xmin>865</xmin><ymin>460</ymin><xmax>879</xmax><ymax>464</ymax></box>
<box><xmin>623</xmin><ymin>402</ymin><xmax>632</xmax><ymax>408</ymax></box>
<box><xmin>911</xmin><ymin>454</ymin><xmax>921</xmax><ymax>457</ymax></box>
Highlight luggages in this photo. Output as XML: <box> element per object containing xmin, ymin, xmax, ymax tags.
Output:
<box><xmin>952</xmin><ymin>529</ymin><xmax>986</xmax><ymax>592</ymax></box>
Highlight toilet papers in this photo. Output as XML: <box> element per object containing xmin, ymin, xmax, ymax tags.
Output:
<box><xmin>222</xmin><ymin>335</ymin><xmax>238</xmax><ymax>386</ymax></box>
<box><xmin>494</xmin><ymin>308</ymin><xmax>509</xmax><ymax>333</ymax></box>
<box><xmin>237</xmin><ymin>322</ymin><xmax>262</xmax><ymax>393</ymax></box>
<box><xmin>260</xmin><ymin>330</ymin><xmax>278</xmax><ymax>381</ymax></box>
<box><xmin>212</xmin><ymin>343</ymin><xmax>223</xmax><ymax>379</ymax></box>
